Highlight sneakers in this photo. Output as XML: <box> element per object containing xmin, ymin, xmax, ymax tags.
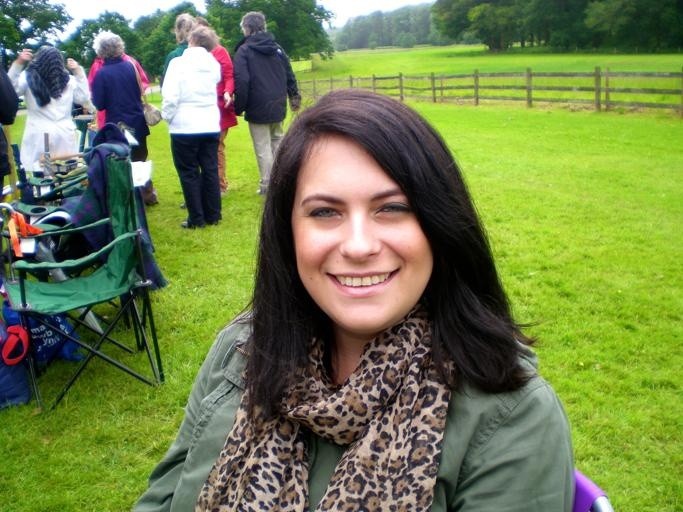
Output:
<box><xmin>180</xmin><ymin>187</ymin><xmax>227</xmax><ymax>227</ymax></box>
<box><xmin>142</xmin><ymin>191</ymin><xmax>157</xmax><ymax>207</ymax></box>
<box><xmin>256</xmin><ymin>188</ymin><xmax>266</xmax><ymax>194</ymax></box>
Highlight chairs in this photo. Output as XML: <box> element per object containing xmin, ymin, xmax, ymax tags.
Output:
<box><xmin>4</xmin><ymin>145</ymin><xmax>165</xmax><ymax>414</ymax></box>
<box><xmin>572</xmin><ymin>463</ymin><xmax>611</xmax><ymax>512</ymax></box>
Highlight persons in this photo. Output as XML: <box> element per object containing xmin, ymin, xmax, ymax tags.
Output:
<box><xmin>162</xmin><ymin>13</ymin><xmax>196</xmax><ymax>208</ymax></box>
<box><xmin>88</xmin><ymin>31</ymin><xmax>159</xmax><ymax>207</ymax></box>
<box><xmin>161</xmin><ymin>26</ymin><xmax>221</xmax><ymax>230</ymax></box>
<box><xmin>196</xmin><ymin>16</ymin><xmax>238</xmax><ymax>193</ymax></box>
<box><xmin>0</xmin><ymin>68</ymin><xmax>20</xmax><ymax>184</ymax></box>
<box><xmin>9</xmin><ymin>45</ymin><xmax>92</xmax><ymax>178</ymax></box>
<box><xmin>119</xmin><ymin>84</ymin><xmax>579</xmax><ymax>510</ymax></box>
<box><xmin>232</xmin><ymin>12</ymin><xmax>301</xmax><ymax>193</ymax></box>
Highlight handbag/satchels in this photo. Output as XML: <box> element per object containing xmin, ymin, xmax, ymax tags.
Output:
<box><xmin>141</xmin><ymin>102</ymin><xmax>162</xmax><ymax>127</ymax></box>
<box><xmin>0</xmin><ymin>323</ymin><xmax>31</xmax><ymax>410</ymax></box>
<box><xmin>1</xmin><ymin>299</ymin><xmax>83</xmax><ymax>364</ymax></box>
